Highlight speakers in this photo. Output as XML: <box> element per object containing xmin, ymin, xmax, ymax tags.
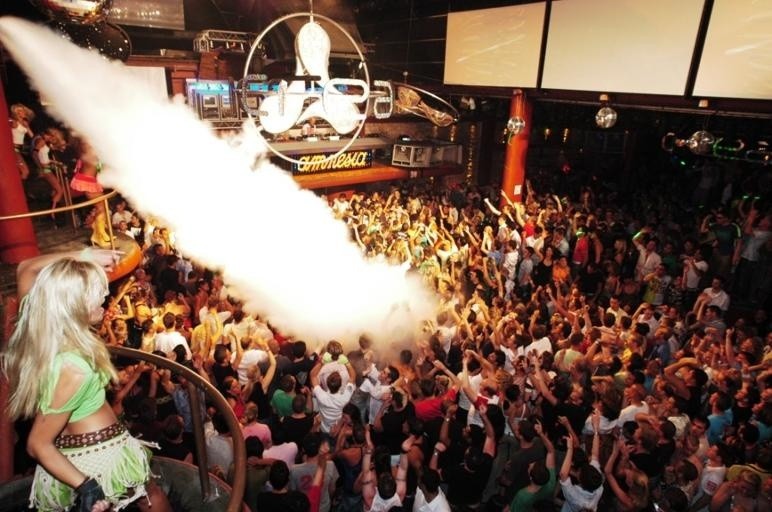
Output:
<box><xmin>391</xmin><ymin>138</ymin><xmax>463</xmax><ymax>168</ymax></box>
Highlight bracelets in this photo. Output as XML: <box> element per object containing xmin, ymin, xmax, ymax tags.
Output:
<box><xmin>74</xmin><ymin>475</ymin><xmax>91</xmax><ymax>492</ymax></box>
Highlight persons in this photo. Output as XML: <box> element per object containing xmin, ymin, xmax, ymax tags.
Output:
<box><xmin>32</xmin><ymin>128</ymin><xmax>67</xmax><ymax>219</ymax></box>
<box><xmin>9</xmin><ymin>104</ymin><xmax>36</xmax><ymax>180</ymax></box>
<box><xmin>0</xmin><ymin>247</ymin><xmax>170</xmax><ymax>512</ymax></box>
<box><xmin>71</xmin><ymin>138</ymin><xmax>772</xmax><ymax>512</ymax></box>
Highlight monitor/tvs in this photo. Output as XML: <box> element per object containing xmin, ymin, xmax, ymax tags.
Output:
<box><xmin>240</xmin><ymin>96</ymin><xmax>258</xmax><ymax>109</ymax></box>
<box><xmin>203</xmin><ymin>95</ymin><xmax>218</xmax><ymax>108</ymax></box>
